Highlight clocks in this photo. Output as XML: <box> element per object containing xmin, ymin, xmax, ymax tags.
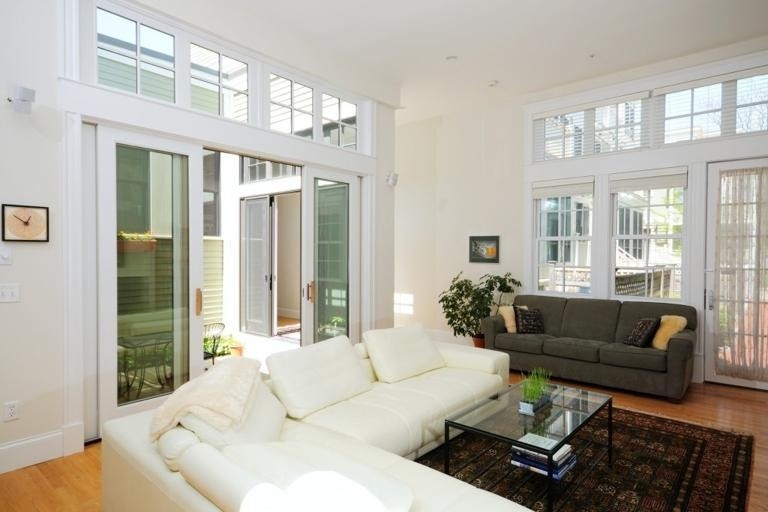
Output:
<box><xmin>2</xmin><ymin>204</ymin><xmax>49</xmax><ymax>242</ymax></box>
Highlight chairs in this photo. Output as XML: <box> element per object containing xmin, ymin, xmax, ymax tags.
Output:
<box><xmin>203</xmin><ymin>323</ymin><xmax>226</xmax><ymax>367</ymax></box>
<box><xmin>118</xmin><ymin>355</ymin><xmax>138</xmax><ymax>399</ymax></box>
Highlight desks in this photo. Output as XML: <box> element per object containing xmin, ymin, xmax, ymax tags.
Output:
<box><xmin>117</xmin><ymin>330</ymin><xmax>174</xmax><ymax>401</ymax></box>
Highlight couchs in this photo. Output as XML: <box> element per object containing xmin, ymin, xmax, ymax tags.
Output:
<box><xmin>484</xmin><ymin>294</ymin><xmax>697</xmax><ymax>404</ymax></box>
<box><xmin>101</xmin><ymin>321</ymin><xmax>545</xmax><ymax>511</ymax></box>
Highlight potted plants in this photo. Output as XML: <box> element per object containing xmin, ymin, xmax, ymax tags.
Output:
<box><xmin>438</xmin><ymin>269</ymin><xmax>522</xmax><ymax>348</ymax></box>
<box><xmin>517</xmin><ymin>367</ymin><xmax>553</xmax><ymax>417</ymax></box>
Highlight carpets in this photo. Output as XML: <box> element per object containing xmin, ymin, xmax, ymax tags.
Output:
<box><xmin>414</xmin><ymin>387</ymin><xmax>755</xmax><ymax>512</ymax></box>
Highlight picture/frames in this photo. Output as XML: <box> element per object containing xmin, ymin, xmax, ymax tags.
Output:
<box><xmin>469</xmin><ymin>236</ymin><xmax>500</xmax><ymax>263</ymax></box>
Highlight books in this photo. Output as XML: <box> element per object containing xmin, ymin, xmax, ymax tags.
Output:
<box><xmin>510</xmin><ymin>432</ymin><xmax>577</xmax><ymax>480</ymax></box>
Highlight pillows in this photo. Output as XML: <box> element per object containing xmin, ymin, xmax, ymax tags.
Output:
<box><xmin>653</xmin><ymin>315</ymin><xmax>688</xmax><ymax>349</ymax></box>
<box><xmin>178</xmin><ymin>378</ymin><xmax>287</xmax><ymax>450</ymax></box>
<box><xmin>363</xmin><ymin>322</ymin><xmax>447</xmax><ymax>384</ymax></box>
<box><xmin>499</xmin><ymin>305</ymin><xmax>516</xmax><ymax>334</ymax></box>
<box><xmin>266</xmin><ymin>335</ymin><xmax>376</xmax><ymax>420</ymax></box>
<box><xmin>624</xmin><ymin>317</ymin><xmax>660</xmax><ymax>348</ymax></box>
<box><xmin>513</xmin><ymin>305</ymin><xmax>547</xmax><ymax>335</ymax></box>
